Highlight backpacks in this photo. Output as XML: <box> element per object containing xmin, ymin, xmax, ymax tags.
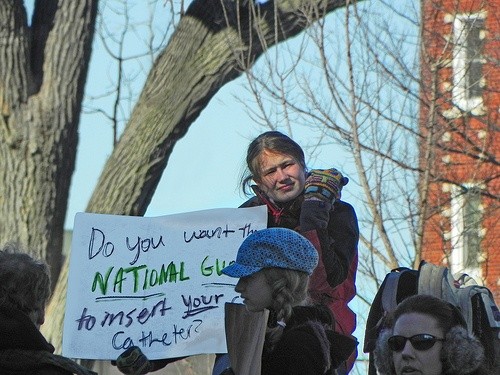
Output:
<box><xmin>454</xmin><ymin>272</ymin><xmax>500</xmax><ymax>364</ymax></box>
<box><xmin>363</xmin><ymin>260</ymin><xmax>458</xmax><ymax>375</ymax></box>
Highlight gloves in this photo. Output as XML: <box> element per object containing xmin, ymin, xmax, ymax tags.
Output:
<box><xmin>303</xmin><ymin>168</ymin><xmax>349</xmax><ymax>205</ymax></box>
<box><xmin>111</xmin><ymin>346</ymin><xmax>168</xmax><ymax>375</ymax></box>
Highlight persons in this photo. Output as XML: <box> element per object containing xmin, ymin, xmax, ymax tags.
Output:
<box><xmin>1</xmin><ymin>242</ymin><xmax>101</xmax><ymax>375</ymax></box>
<box><xmin>212</xmin><ymin>227</ymin><xmax>332</xmax><ymax>375</ymax></box>
<box><xmin>388</xmin><ymin>295</ymin><xmax>467</xmax><ymax>373</ymax></box>
<box><xmin>113</xmin><ymin>132</ymin><xmax>359</xmax><ymax>375</ymax></box>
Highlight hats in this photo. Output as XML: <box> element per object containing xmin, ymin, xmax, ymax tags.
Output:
<box><xmin>221</xmin><ymin>228</ymin><xmax>320</xmax><ymax>278</ymax></box>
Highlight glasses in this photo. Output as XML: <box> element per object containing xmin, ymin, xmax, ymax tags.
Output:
<box><xmin>388</xmin><ymin>333</ymin><xmax>446</xmax><ymax>351</ymax></box>
